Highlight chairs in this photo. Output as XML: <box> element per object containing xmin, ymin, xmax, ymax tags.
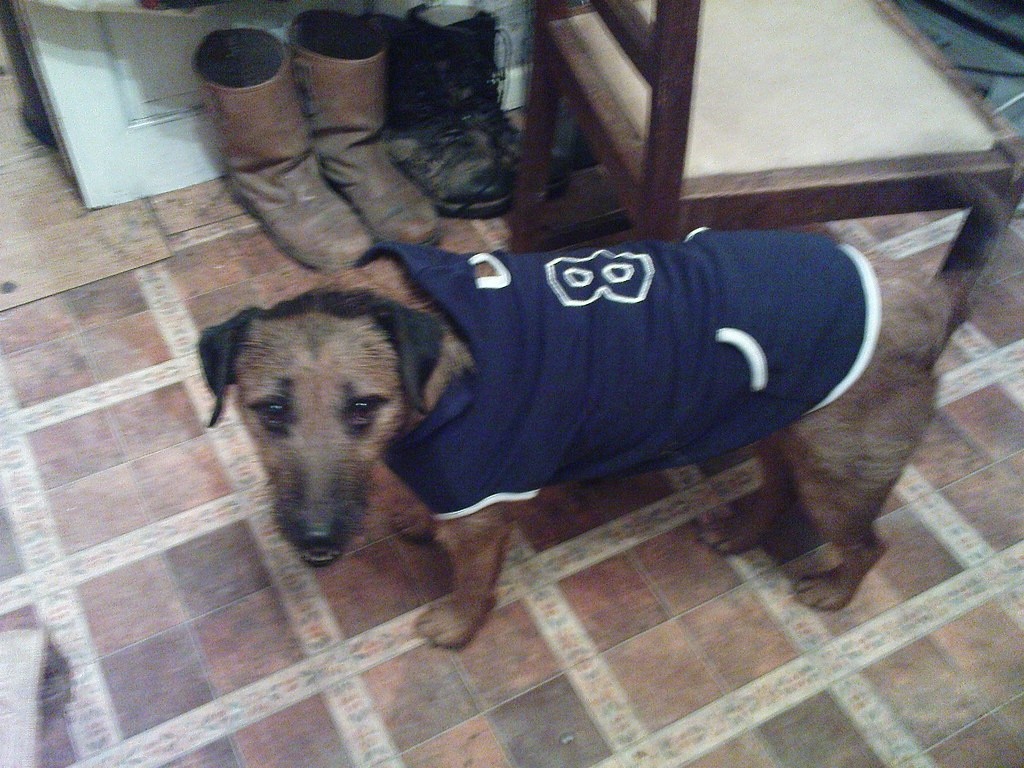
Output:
<box><xmin>508</xmin><ymin>0</ymin><xmax>1024</xmax><ymax>299</ymax></box>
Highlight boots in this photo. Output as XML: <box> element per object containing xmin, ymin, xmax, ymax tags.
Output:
<box><xmin>192</xmin><ymin>27</ymin><xmax>374</xmax><ymax>268</ymax></box>
<box><xmin>366</xmin><ymin>12</ymin><xmax>512</xmax><ymax>216</ymax></box>
<box><xmin>286</xmin><ymin>7</ymin><xmax>441</xmax><ymax>245</ymax></box>
<box><xmin>407</xmin><ymin>2</ymin><xmax>522</xmax><ymax>175</ymax></box>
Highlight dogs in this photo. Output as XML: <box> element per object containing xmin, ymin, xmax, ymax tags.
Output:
<box><xmin>193</xmin><ymin>174</ymin><xmax>1006</xmax><ymax>653</ymax></box>
